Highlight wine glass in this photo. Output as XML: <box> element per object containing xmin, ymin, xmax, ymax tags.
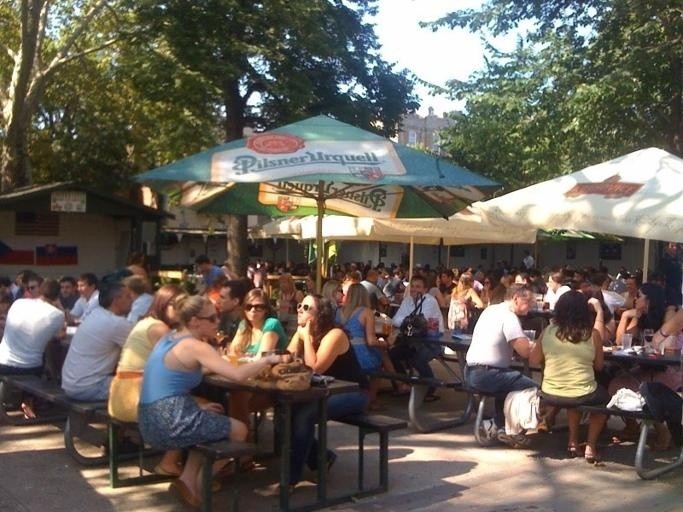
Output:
<box><xmin>644</xmin><ymin>329</ymin><xmax>654</xmax><ymax>353</ymax></box>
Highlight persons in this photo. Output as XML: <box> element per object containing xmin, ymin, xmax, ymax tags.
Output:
<box><xmin>0</xmin><ymin>250</ymin><xmax>682</xmax><ymax>512</ymax></box>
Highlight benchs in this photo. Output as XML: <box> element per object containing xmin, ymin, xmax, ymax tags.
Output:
<box><xmin>330</xmin><ymin>409</ymin><xmax>408</xmax><ymax>503</ymax></box>
<box><xmin>0</xmin><ymin>374</ymin><xmax>257</xmax><ymax>511</ymax></box>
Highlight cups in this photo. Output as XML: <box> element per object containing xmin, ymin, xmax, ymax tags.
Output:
<box><xmin>228</xmin><ymin>354</ymin><xmax>239</xmax><ymax>367</ymax></box>
<box><xmin>622</xmin><ymin>334</ymin><xmax>633</xmax><ymax>349</ymax></box>
<box><xmin>523</xmin><ymin>330</ymin><xmax>536</xmax><ymax>342</ymax></box>
<box><xmin>537</xmin><ymin>302</ymin><xmax>543</xmax><ymax>311</ymax></box>
<box><xmin>454</xmin><ymin>320</ymin><xmax>461</xmax><ymax>329</ymax></box>
<box><xmin>381</xmin><ymin>320</ymin><xmax>392</xmax><ymax>333</ymax></box>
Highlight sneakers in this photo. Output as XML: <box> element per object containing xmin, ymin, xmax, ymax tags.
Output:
<box><xmin>537</xmin><ymin>422</ymin><xmax>549</xmax><ymax>432</ymax></box>
<box><xmin>482</xmin><ymin>419</ymin><xmax>500</xmax><ymax>441</ymax></box>
<box><xmin>424</xmin><ymin>389</ymin><xmax>440</xmax><ymax>402</ymax></box>
<box><xmin>274</xmin><ymin>481</ymin><xmax>293</xmax><ymax>496</ymax></box>
<box><xmin>394</xmin><ymin>384</ymin><xmax>410</xmax><ymax>395</ymax></box>
<box><xmin>20</xmin><ymin>399</ymin><xmax>39</xmax><ymax>419</ymax></box>
<box><xmin>313</xmin><ymin>453</ymin><xmax>336</xmax><ymax>483</ymax></box>
<box><xmin>153</xmin><ymin>463</ymin><xmax>183</xmax><ymax>477</ymax></box>
<box><xmin>497</xmin><ymin>426</ymin><xmax>530</xmax><ymax>448</ymax></box>
<box><xmin>168</xmin><ymin>480</ymin><xmax>201</xmax><ymax>509</ymax></box>
<box><xmin>221</xmin><ymin>454</ymin><xmax>254</xmax><ymax>477</ymax></box>
<box><xmin>211</xmin><ymin>482</ymin><xmax>220</xmax><ymax>492</ymax></box>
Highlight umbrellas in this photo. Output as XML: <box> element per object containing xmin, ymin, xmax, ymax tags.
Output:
<box><xmin>129</xmin><ymin>114</ymin><xmax>503</xmax><ymax>296</ymax></box>
<box><xmin>454</xmin><ymin>147</ymin><xmax>683</xmax><ymax>283</ymax></box>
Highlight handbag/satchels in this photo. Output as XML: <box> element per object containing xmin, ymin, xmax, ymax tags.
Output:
<box><xmin>400</xmin><ymin>314</ymin><xmax>427</xmax><ymax>338</ymax></box>
<box><xmin>640</xmin><ymin>382</ymin><xmax>682</xmax><ymax>417</ymax></box>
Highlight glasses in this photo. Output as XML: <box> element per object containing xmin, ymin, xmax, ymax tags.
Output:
<box><xmin>245</xmin><ymin>303</ymin><xmax>267</xmax><ymax>312</ymax></box>
<box><xmin>297</xmin><ymin>303</ymin><xmax>318</xmax><ymax>312</ymax></box>
<box><xmin>197</xmin><ymin>314</ymin><xmax>217</xmax><ymax>323</ymax></box>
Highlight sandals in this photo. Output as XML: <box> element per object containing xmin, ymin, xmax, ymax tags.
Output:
<box><xmin>565</xmin><ymin>442</ymin><xmax>601</xmax><ymax>461</ymax></box>
